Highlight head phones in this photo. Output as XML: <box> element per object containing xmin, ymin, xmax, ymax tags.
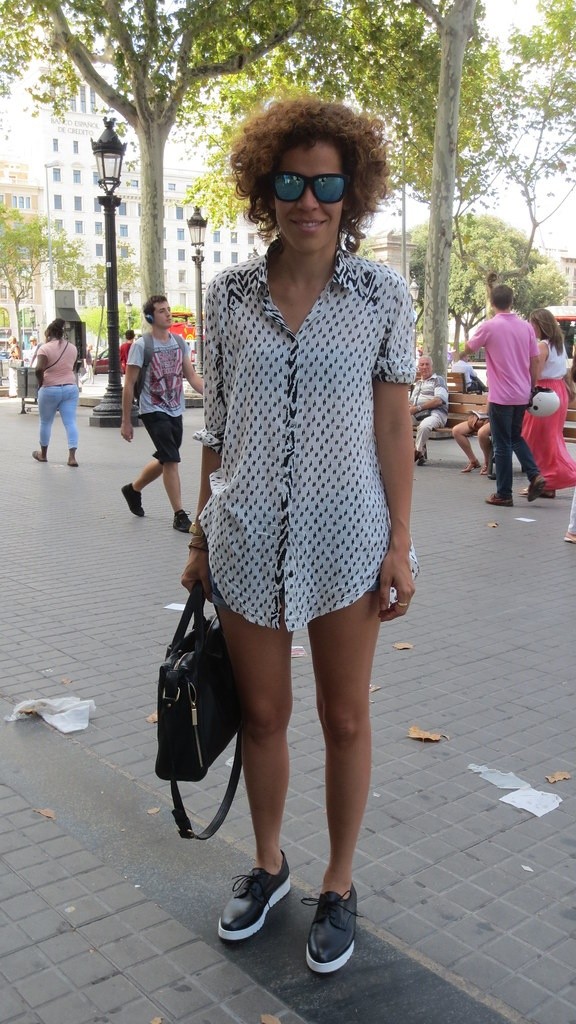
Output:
<box><xmin>145</xmin><ymin>314</ymin><xmax>154</xmax><ymax>324</ymax></box>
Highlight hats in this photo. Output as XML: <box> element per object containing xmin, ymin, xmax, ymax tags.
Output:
<box><xmin>29</xmin><ymin>335</ymin><xmax>38</xmax><ymax>342</ymax></box>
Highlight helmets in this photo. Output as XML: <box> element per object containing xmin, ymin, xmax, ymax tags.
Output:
<box><xmin>527</xmin><ymin>385</ymin><xmax>560</xmax><ymax>417</ymax></box>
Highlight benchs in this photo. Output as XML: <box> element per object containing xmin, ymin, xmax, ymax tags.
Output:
<box><xmin>410</xmin><ymin>373</ymin><xmax>576</xmax><ymax>480</ymax></box>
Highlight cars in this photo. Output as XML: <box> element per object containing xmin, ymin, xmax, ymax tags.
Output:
<box><xmin>22</xmin><ymin>350</ymin><xmax>38</xmax><ymax>367</ymax></box>
<box><xmin>92</xmin><ymin>344</ymin><xmax>109</xmax><ymax>375</ymax></box>
<box><xmin>0</xmin><ymin>351</ymin><xmax>9</xmax><ymax>360</ymax></box>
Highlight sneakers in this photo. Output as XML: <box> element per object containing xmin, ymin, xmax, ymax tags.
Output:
<box><xmin>301</xmin><ymin>883</ymin><xmax>363</xmax><ymax>974</ymax></box>
<box><xmin>172</xmin><ymin>510</ymin><xmax>193</xmax><ymax>533</ymax></box>
<box><xmin>217</xmin><ymin>850</ymin><xmax>291</xmax><ymax>940</ymax></box>
<box><xmin>121</xmin><ymin>483</ymin><xmax>145</xmax><ymax>517</ymax></box>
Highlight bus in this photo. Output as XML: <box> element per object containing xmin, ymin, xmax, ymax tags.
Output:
<box><xmin>168</xmin><ymin>311</ymin><xmax>206</xmax><ymax>340</ymax></box>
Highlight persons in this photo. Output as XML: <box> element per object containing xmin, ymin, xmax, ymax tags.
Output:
<box><xmin>118</xmin><ymin>294</ymin><xmax>204</xmax><ymax>533</ymax></box>
<box><xmin>32</xmin><ymin>316</ymin><xmax>81</xmax><ymax>467</ymax></box>
<box><xmin>459</xmin><ymin>284</ymin><xmax>546</xmax><ymax>507</ymax></box>
<box><xmin>80</xmin><ymin>343</ymin><xmax>95</xmax><ymax>387</ymax></box>
<box><xmin>180</xmin><ymin>97</ymin><xmax>415</xmax><ymax>977</ymax></box>
<box><xmin>6</xmin><ymin>334</ymin><xmax>39</xmax><ymax>399</ymax></box>
<box><xmin>520</xmin><ymin>308</ymin><xmax>576</xmax><ymax>544</ymax></box>
<box><xmin>409</xmin><ymin>354</ymin><xmax>449</xmax><ymax>466</ymax></box>
<box><xmin>451</xmin><ymin>351</ymin><xmax>490</xmax><ymax>476</ymax></box>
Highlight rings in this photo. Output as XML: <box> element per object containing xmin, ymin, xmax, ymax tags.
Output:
<box><xmin>394</xmin><ymin>601</ymin><xmax>408</xmax><ymax>608</ymax></box>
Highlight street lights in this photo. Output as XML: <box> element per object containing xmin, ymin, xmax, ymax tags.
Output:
<box><xmin>90</xmin><ymin>118</ymin><xmax>143</xmax><ymax>427</ymax></box>
<box><xmin>28</xmin><ymin>306</ymin><xmax>35</xmax><ymax>329</ymax></box>
<box><xmin>410</xmin><ymin>279</ymin><xmax>419</xmax><ymax>360</ymax></box>
<box><xmin>124</xmin><ymin>299</ymin><xmax>133</xmax><ymax>331</ymax></box>
<box><xmin>185</xmin><ymin>206</ymin><xmax>208</xmax><ymax>409</ymax></box>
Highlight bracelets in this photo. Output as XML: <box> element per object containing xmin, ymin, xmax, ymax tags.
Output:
<box><xmin>416</xmin><ymin>404</ymin><xmax>423</xmax><ymax>413</ymax></box>
<box><xmin>187</xmin><ymin>536</ymin><xmax>208</xmax><ymax>552</ymax></box>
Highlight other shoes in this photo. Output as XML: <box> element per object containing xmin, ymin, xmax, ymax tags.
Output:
<box><xmin>518</xmin><ymin>487</ymin><xmax>555</xmax><ymax>498</ymax></box>
<box><xmin>527</xmin><ymin>475</ymin><xmax>546</xmax><ymax>502</ymax></box>
<box><xmin>32</xmin><ymin>451</ymin><xmax>48</xmax><ymax>462</ymax></box>
<box><xmin>480</xmin><ymin>462</ymin><xmax>487</xmax><ymax>474</ymax></box>
<box><xmin>564</xmin><ymin>532</ymin><xmax>576</xmax><ymax>544</ymax></box>
<box><xmin>417</xmin><ymin>455</ymin><xmax>426</xmax><ymax>466</ymax></box>
<box><xmin>487</xmin><ymin>472</ymin><xmax>496</xmax><ymax>480</ymax></box>
<box><xmin>485</xmin><ymin>493</ymin><xmax>513</xmax><ymax>506</ymax></box>
<box><xmin>414</xmin><ymin>449</ymin><xmax>422</xmax><ymax>462</ymax></box>
<box><xmin>67</xmin><ymin>457</ymin><xmax>78</xmax><ymax>467</ymax></box>
<box><xmin>461</xmin><ymin>459</ymin><xmax>481</xmax><ymax>472</ymax></box>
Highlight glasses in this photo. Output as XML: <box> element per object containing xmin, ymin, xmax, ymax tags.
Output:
<box><xmin>270</xmin><ymin>171</ymin><xmax>346</xmax><ymax>204</ymax></box>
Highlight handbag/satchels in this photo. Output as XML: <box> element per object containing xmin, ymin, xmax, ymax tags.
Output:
<box><xmin>468</xmin><ymin>414</ymin><xmax>489</xmax><ymax>431</ymax></box>
<box><xmin>413</xmin><ymin>410</ymin><xmax>431</xmax><ymax>421</ymax></box>
<box><xmin>8</xmin><ymin>357</ymin><xmax>24</xmax><ymax>370</ymax></box>
<box><xmin>155</xmin><ymin>582</ymin><xmax>242</xmax><ymax>840</ymax></box>
